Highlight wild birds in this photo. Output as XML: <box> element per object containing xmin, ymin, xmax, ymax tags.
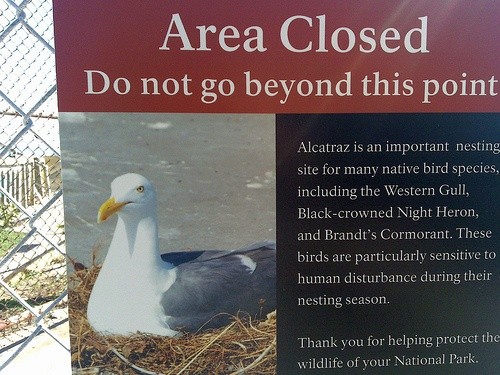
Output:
<box><xmin>87</xmin><ymin>173</ymin><xmax>276</xmax><ymax>339</ymax></box>
